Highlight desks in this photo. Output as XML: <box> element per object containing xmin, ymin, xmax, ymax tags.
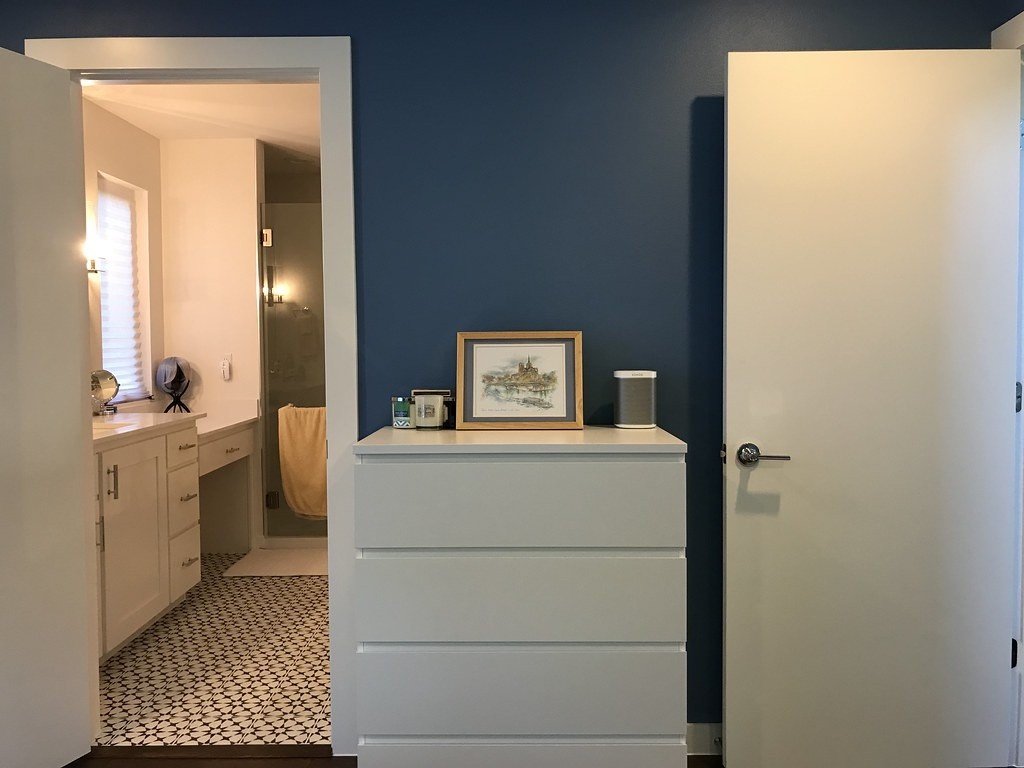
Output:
<box><xmin>354</xmin><ymin>426</ymin><xmax>689</xmax><ymax>768</ymax></box>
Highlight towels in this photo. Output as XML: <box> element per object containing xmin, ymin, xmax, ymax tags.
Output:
<box><xmin>278</xmin><ymin>403</ymin><xmax>327</xmax><ymax>521</ymax></box>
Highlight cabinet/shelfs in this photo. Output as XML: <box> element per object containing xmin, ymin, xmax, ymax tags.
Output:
<box><xmin>93</xmin><ymin>412</ymin><xmax>256</xmax><ymax>666</ymax></box>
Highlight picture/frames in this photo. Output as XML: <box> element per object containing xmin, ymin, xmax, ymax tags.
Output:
<box><xmin>456</xmin><ymin>330</ymin><xmax>584</xmax><ymax>429</ymax></box>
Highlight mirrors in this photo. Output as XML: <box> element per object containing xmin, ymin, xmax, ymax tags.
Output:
<box><xmin>91</xmin><ymin>369</ymin><xmax>120</xmax><ymax>413</ymax></box>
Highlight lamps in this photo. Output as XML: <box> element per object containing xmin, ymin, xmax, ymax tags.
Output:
<box><xmin>265</xmin><ymin>265</ymin><xmax>284</xmax><ymax>306</ymax></box>
<box><xmin>85</xmin><ymin>235</ymin><xmax>105</xmax><ymax>273</ymax></box>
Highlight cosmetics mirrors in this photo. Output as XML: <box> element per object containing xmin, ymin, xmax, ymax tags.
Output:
<box><xmin>90</xmin><ymin>370</ymin><xmax>120</xmax><ymax>415</ymax></box>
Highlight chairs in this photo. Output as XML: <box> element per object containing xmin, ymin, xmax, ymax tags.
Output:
<box><xmin>276</xmin><ymin>403</ymin><xmax>327</xmax><ymax>523</ymax></box>
